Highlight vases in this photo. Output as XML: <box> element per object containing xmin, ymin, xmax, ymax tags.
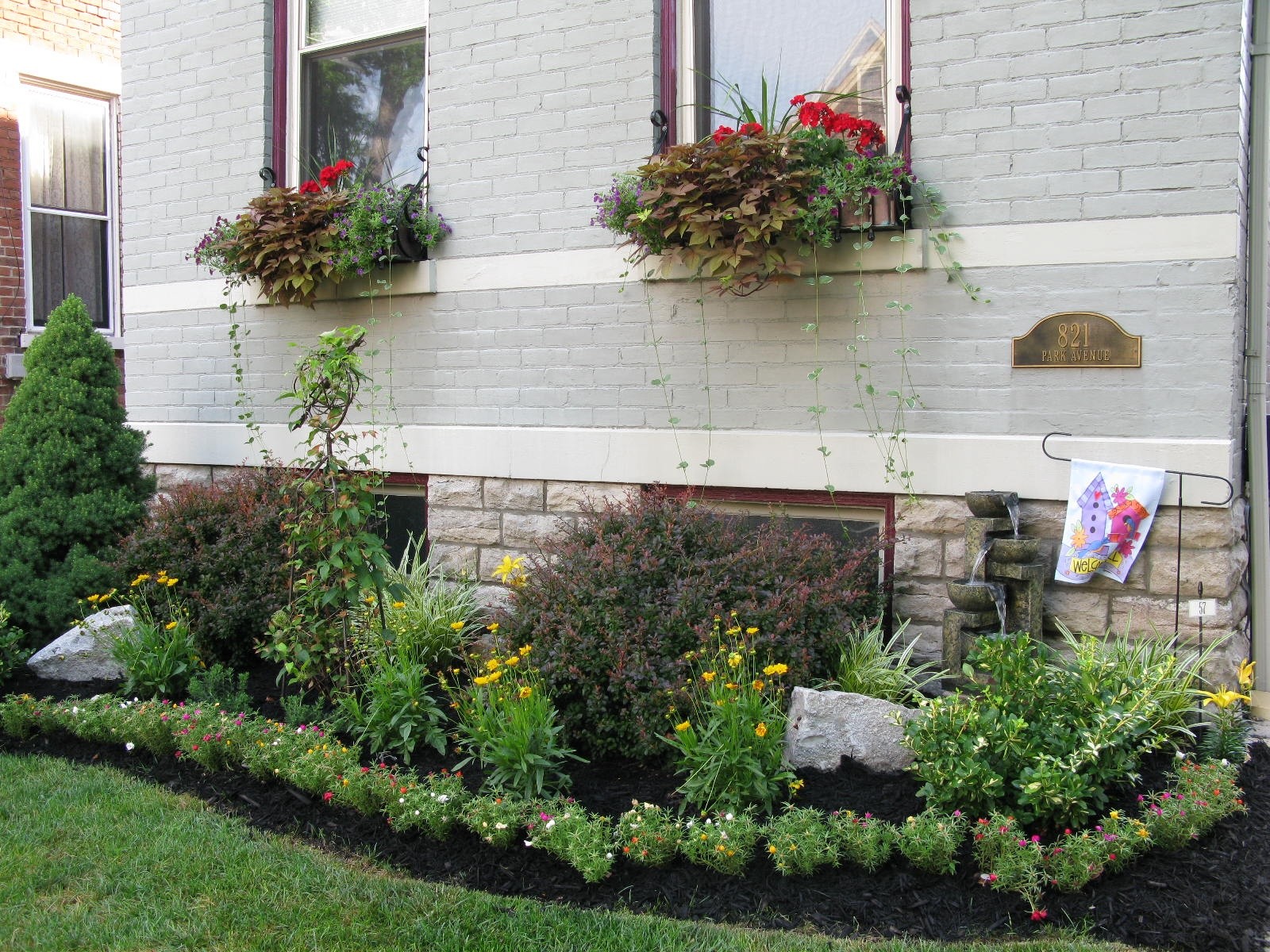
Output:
<box><xmin>371</xmin><ymin>210</ymin><xmax>426</xmax><ymax>264</ymax></box>
<box><xmin>826</xmin><ymin>183</ymin><xmax>903</xmax><ymax>230</ymax></box>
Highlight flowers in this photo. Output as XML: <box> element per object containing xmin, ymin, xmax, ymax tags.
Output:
<box><xmin>588</xmin><ymin>46</ymin><xmax>991</xmax><ymax>552</ymax></box>
<box><xmin>180</xmin><ymin>155</ymin><xmax>464</xmax><ymax>539</ymax></box>
